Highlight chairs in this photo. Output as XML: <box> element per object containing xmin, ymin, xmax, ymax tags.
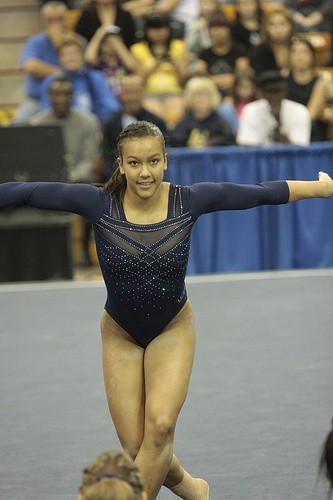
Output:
<box><xmin>0</xmin><ymin>123</ymin><xmax>73</xmax><ymax>286</ymax></box>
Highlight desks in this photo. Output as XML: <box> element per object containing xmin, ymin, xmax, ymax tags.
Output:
<box><xmin>162</xmin><ymin>143</ymin><xmax>333</xmax><ymax>276</ymax></box>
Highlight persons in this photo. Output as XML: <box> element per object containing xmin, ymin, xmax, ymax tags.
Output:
<box><xmin>77</xmin><ymin>450</ymin><xmax>147</xmax><ymax>500</ymax></box>
<box><xmin>315</xmin><ymin>420</ymin><xmax>333</xmax><ymax>500</ymax></box>
<box><xmin>17</xmin><ymin>0</ymin><xmax>333</xmax><ymax>142</ymax></box>
<box><xmin>29</xmin><ymin>78</ymin><xmax>104</xmax><ymax>269</ymax></box>
<box><xmin>0</xmin><ymin>120</ymin><xmax>333</xmax><ymax>500</ymax></box>
